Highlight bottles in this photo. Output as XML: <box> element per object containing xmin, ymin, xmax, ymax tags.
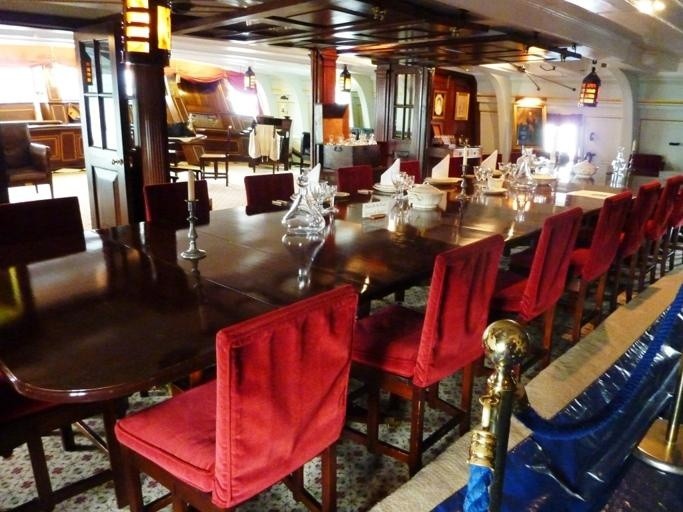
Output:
<box><xmin>281</xmin><ymin>233</ymin><xmax>326</xmax><ymax>291</ymax></box>
<box><xmin>505</xmin><ymin>157</ymin><xmax>539</xmax><ymax>191</ymax></box>
<box><xmin>611</xmin><ymin>144</ymin><xmax>627</xmax><ymax>171</ymax></box>
<box><xmin>281</xmin><ymin>175</ymin><xmax>326</xmax><ymax>235</ymax></box>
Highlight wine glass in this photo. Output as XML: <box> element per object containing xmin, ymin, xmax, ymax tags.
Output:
<box><xmin>472</xmin><ymin>164</ymin><xmax>493</xmax><ymax>191</ymax></box>
<box><xmin>328</xmin><ymin>132</ymin><xmax>375</xmax><ymax>145</ymax></box>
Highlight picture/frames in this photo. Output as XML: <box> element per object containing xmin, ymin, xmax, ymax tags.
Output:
<box><xmin>432</xmin><ymin>90</ymin><xmax>447</xmax><ymax>120</ymax></box>
<box><xmin>453</xmin><ymin>92</ymin><xmax>470</xmax><ymax>120</ymax></box>
<box><xmin>511</xmin><ymin>102</ymin><xmax>548</xmax><ymax>150</ymax></box>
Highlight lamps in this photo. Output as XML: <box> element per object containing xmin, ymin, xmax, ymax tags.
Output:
<box><xmin>337</xmin><ymin>64</ymin><xmax>351</xmax><ymax>93</ymax></box>
<box><xmin>577</xmin><ymin>57</ymin><xmax>601</xmax><ymax>108</ymax></box>
<box><xmin>242</xmin><ymin>66</ymin><xmax>257</xmax><ymax>93</ymax></box>
<box><xmin>119</xmin><ymin>0</ymin><xmax>172</xmax><ymax>66</ymax></box>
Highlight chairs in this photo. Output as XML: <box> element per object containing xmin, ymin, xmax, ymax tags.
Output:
<box><xmin>0</xmin><ymin>123</ymin><xmax>683</xmax><ymax>511</ymax></box>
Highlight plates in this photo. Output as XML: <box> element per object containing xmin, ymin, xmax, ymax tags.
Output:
<box><xmin>333</xmin><ymin>173</ymin><xmax>557</xmax><ymax>197</ymax></box>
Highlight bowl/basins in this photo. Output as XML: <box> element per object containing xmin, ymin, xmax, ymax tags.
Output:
<box><xmin>571</xmin><ymin>160</ymin><xmax>600</xmax><ymax>178</ymax></box>
<box><xmin>406</xmin><ymin>180</ymin><xmax>443</xmax><ymax>210</ymax></box>
